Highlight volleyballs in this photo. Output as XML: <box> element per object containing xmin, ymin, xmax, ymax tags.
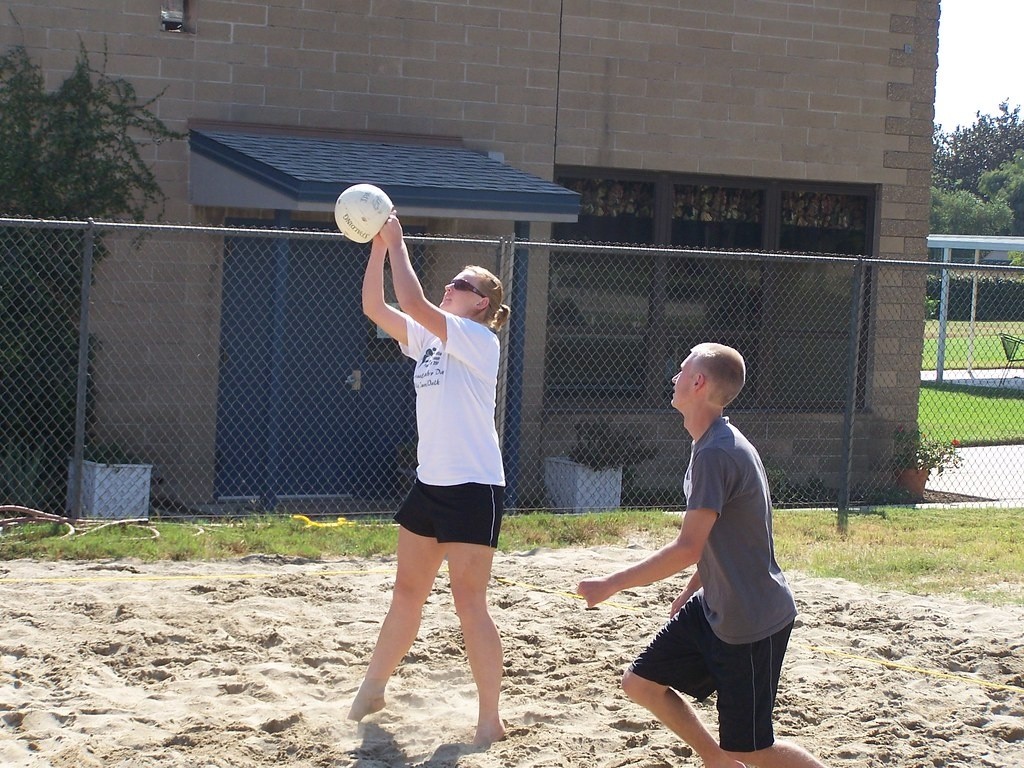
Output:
<box><xmin>333</xmin><ymin>183</ymin><xmax>396</xmax><ymax>244</ymax></box>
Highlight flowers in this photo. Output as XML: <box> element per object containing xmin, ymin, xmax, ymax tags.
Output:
<box><xmin>890</xmin><ymin>426</ymin><xmax>965</xmax><ymax>477</ymax></box>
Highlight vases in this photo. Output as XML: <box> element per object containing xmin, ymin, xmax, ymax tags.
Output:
<box><xmin>895</xmin><ymin>467</ymin><xmax>929</xmax><ymax>496</ymax></box>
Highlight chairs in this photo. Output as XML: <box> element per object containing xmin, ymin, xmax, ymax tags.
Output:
<box><xmin>998</xmin><ymin>333</ymin><xmax>1024</xmax><ymax>387</ymax></box>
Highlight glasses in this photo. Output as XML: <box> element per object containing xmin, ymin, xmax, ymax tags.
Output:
<box><xmin>450</xmin><ymin>279</ymin><xmax>493</xmax><ymax>307</ymax></box>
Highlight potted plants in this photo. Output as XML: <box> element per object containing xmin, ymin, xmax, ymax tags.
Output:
<box><xmin>543</xmin><ymin>418</ymin><xmax>660</xmax><ymax>513</ymax></box>
<box><xmin>66</xmin><ymin>444</ymin><xmax>153</xmax><ymax>518</ymax></box>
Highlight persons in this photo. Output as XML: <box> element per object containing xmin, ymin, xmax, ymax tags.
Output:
<box><xmin>346</xmin><ymin>210</ymin><xmax>512</xmax><ymax>743</ymax></box>
<box><xmin>576</xmin><ymin>343</ymin><xmax>826</xmax><ymax>768</ymax></box>
